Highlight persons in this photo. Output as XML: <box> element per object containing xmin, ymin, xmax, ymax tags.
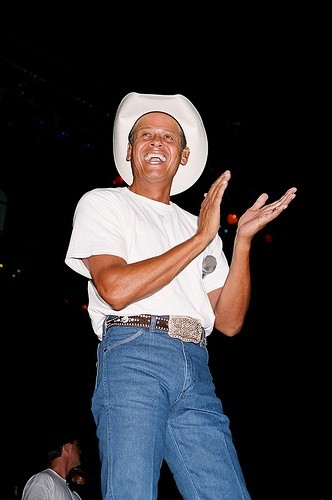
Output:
<box><xmin>21</xmin><ymin>436</ymin><xmax>82</xmax><ymax>500</ymax></box>
<box><xmin>65</xmin><ymin>92</ymin><xmax>297</xmax><ymax>500</ymax></box>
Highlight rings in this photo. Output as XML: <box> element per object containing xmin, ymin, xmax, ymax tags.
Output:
<box><xmin>272</xmin><ymin>208</ymin><xmax>276</xmax><ymax>212</ymax></box>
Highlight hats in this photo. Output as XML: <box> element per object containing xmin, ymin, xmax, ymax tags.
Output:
<box><xmin>113</xmin><ymin>92</ymin><xmax>208</xmax><ymax>196</ymax></box>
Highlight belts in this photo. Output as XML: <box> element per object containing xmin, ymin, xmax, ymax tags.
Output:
<box><xmin>103</xmin><ymin>315</ymin><xmax>206</xmax><ymax>346</ymax></box>
